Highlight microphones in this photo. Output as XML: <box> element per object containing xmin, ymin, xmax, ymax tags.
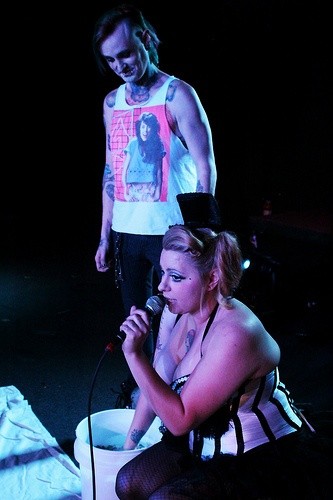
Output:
<box><xmin>106</xmin><ymin>295</ymin><xmax>165</xmax><ymax>352</ymax></box>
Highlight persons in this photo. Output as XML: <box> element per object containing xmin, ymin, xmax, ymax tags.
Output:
<box><xmin>95</xmin><ymin>8</ymin><xmax>217</xmax><ymax>408</ymax></box>
<box><xmin>121</xmin><ymin>112</ymin><xmax>165</xmax><ymax>203</ymax></box>
<box><xmin>115</xmin><ymin>223</ymin><xmax>333</xmax><ymax>500</ymax></box>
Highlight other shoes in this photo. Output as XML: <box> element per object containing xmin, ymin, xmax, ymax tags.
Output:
<box><xmin>119</xmin><ymin>376</ymin><xmax>138</xmax><ymax>392</ymax></box>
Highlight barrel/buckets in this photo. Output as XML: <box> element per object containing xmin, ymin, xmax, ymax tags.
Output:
<box><xmin>73</xmin><ymin>408</ymin><xmax>163</xmax><ymax>500</ymax></box>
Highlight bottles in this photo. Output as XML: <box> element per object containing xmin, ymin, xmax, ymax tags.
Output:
<box><xmin>262</xmin><ymin>200</ymin><xmax>274</xmax><ymax>223</ymax></box>
